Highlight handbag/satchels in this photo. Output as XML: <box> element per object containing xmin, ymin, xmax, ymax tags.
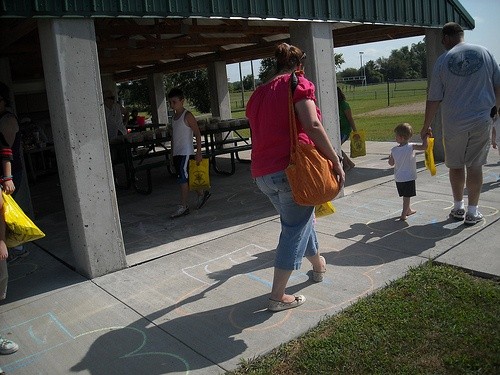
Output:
<box><xmin>315</xmin><ymin>201</ymin><xmax>335</xmax><ymax>218</ymax></box>
<box><xmin>189</xmin><ymin>157</ymin><xmax>211</xmax><ymax>191</ymax></box>
<box><xmin>284</xmin><ymin>69</ymin><xmax>341</xmax><ymax>208</ymax></box>
<box><xmin>424</xmin><ymin>136</ymin><xmax>437</xmax><ymax>176</ymax></box>
<box><xmin>1</xmin><ymin>190</ymin><xmax>46</xmax><ymax>248</ymax></box>
<box><xmin>349</xmin><ymin>130</ymin><xmax>367</xmax><ymax>158</ymax></box>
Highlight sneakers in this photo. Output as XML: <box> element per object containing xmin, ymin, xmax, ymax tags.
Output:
<box><xmin>0</xmin><ymin>338</ymin><xmax>18</xmax><ymax>375</ymax></box>
<box><xmin>451</xmin><ymin>208</ymin><xmax>465</xmax><ymax>219</ymax></box>
<box><xmin>5</xmin><ymin>245</ymin><xmax>30</xmax><ymax>264</ymax></box>
<box><xmin>465</xmin><ymin>212</ymin><xmax>484</xmax><ymax>224</ymax></box>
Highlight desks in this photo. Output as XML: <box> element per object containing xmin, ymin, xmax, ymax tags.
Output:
<box><xmin>112</xmin><ymin>116</ymin><xmax>251</xmax><ymax>187</ymax></box>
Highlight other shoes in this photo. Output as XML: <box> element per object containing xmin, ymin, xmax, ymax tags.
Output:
<box><xmin>313</xmin><ymin>255</ymin><xmax>326</xmax><ymax>283</ymax></box>
<box><xmin>195</xmin><ymin>190</ymin><xmax>212</xmax><ymax>209</ymax></box>
<box><xmin>268</xmin><ymin>294</ymin><xmax>306</xmax><ymax>312</ymax></box>
<box><xmin>171</xmin><ymin>202</ymin><xmax>191</xmax><ymax>218</ymax></box>
<box><xmin>343</xmin><ymin>161</ymin><xmax>355</xmax><ymax>175</ymax></box>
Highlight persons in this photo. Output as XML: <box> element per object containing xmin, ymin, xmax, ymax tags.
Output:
<box><xmin>492</xmin><ymin>109</ymin><xmax>499</xmax><ymax>166</ymax></box>
<box><xmin>0</xmin><ymin>132</ymin><xmax>16</xmax><ymax>303</ymax></box>
<box><xmin>165</xmin><ymin>88</ymin><xmax>213</xmax><ymax>220</ymax></box>
<box><xmin>242</xmin><ymin>43</ymin><xmax>347</xmax><ymax>314</ymax></box>
<box><xmin>336</xmin><ymin>85</ymin><xmax>359</xmax><ymax>174</ymax></box>
<box><xmin>101</xmin><ymin>89</ymin><xmax>136</xmax><ymax>198</ymax></box>
<box><xmin>122</xmin><ymin>106</ymin><xmax>146</xmax><ymax>134</ymax></box>
<box><xmin>14</xmin><ymin>116</ymin><xmax>49</xmax><ymax>151</ymax></box>
<box><xmin>388</xmin><ymin>122</ymin><xmax>431</xmax><ymax>221</ymax></box>
<box><xmin>418</xmin><ymin>21</ymin><xmax>499</xmax><ymax>224</ymax></box>
<box><xmin>0</xmin><ymin>81</ymin><xmax>32</xmax><ymax>268</ymax></box>
<box><xmin>0</xmin><ymin>239</ymin><xmax>18</xmax><ymax>375</ymax></box>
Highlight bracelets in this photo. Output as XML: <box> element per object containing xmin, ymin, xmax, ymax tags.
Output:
<box><xmin>2</xmin><ymin>175</ymin><xmax>13</xmax><ymax>182</ymax></box>
